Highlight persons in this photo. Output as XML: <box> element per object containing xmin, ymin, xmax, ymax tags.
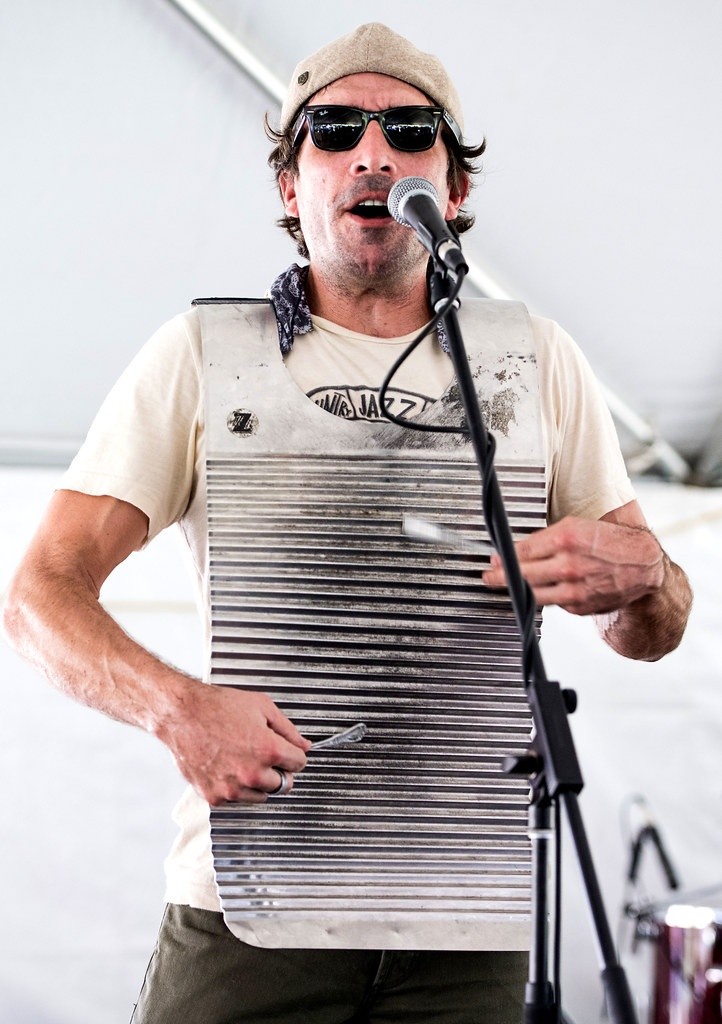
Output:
<box><xmin>1</xmin><ymin>22</ymin><xmax>692</xmax><ymax>1024</ymax></box>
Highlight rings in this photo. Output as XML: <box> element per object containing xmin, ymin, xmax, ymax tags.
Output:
<box><xmin>265</xmin><ymin>767</ymin><xmax>288</xmax><ymax>796</ymax></box>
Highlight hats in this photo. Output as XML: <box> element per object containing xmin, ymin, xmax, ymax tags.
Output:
<box><xmin>280</xmin><ymin>19</ymin><xmax>465</xmax><ymax>138</ymax></box>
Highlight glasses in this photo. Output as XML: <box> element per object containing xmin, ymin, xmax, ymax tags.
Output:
<box><xmin>291</xmin><ymin>103</ymin><xmax>465</xmax><ymax>154</ymax></box>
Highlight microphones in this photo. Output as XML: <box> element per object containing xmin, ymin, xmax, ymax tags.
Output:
<box><xmin>386</xmin><ymin>176</ymin><xmax>468</xmax><ymax>278</ymax></box>
